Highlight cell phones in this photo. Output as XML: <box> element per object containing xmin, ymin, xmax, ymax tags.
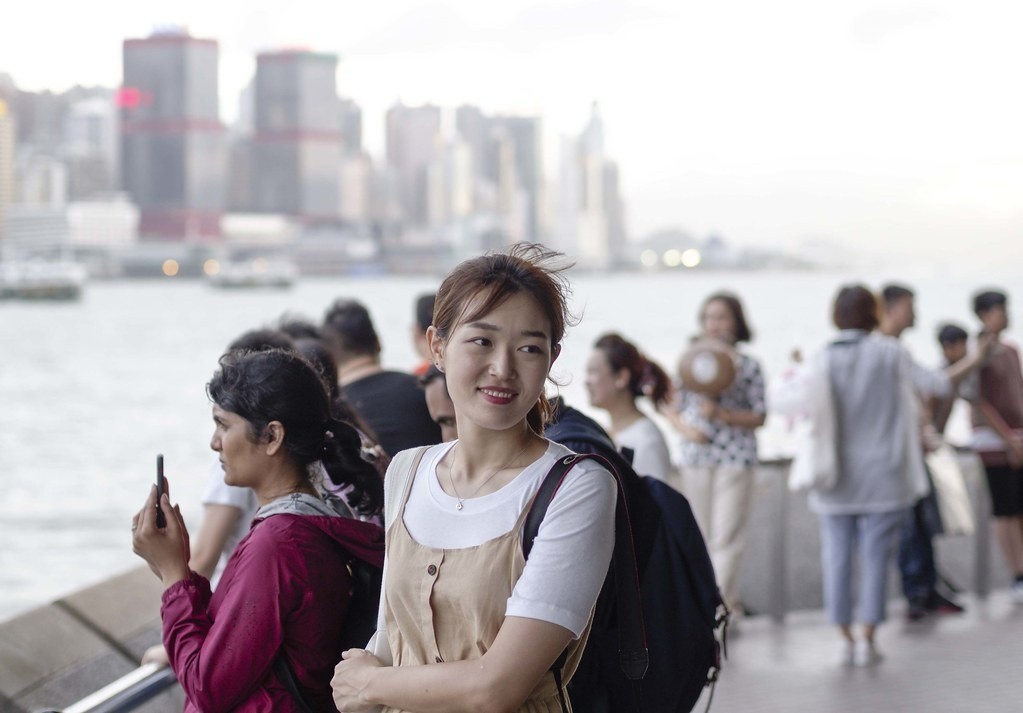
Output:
<box><xmin>156</xmin><ymin>454</ymin><xmax>164</xmax><ymax>526</ymax></box>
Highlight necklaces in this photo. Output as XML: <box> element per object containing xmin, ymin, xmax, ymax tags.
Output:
<box><xmin>439</xmin><ymin>430</ymin><xmax>525</xmax><ymax>516</ymax></box>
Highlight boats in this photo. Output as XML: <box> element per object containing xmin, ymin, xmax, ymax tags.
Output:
<box><xmin>0</xmin><ymin>256</ymin><xmax>84</xmax><ymax>303</ymax></box>
<box><xmin>127</xmin><ymin>243</ymin><xmax>208</xmax><ymax>282</ymax></box>
<box><xmin>193</xmin><ymin>246</ymin><xmax>300</xmax><ymax>285</ymax></box>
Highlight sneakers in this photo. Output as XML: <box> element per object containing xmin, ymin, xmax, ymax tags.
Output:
<box><xmin>832</xmin><ymin>636</ymin><xmax>882</xmax><ymax>666</ymax></box>
<box><xmin>1008</xmin><ymin>579</ymin><xmax>1022</xmax><ymax>603</ymax></box>
<box><xmin>905</xmin><ymin>591</ymin><xmax>964</xmax><ymax>622</ymax></box>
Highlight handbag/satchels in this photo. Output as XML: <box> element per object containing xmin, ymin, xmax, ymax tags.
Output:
<box><xmin>786</xmin><ymin>347</ymin><xmax>843</xmax><ymax>494</ymax></box>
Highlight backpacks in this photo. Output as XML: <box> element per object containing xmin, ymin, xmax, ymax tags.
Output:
<box><xmin>275</xmin><ymin>539</ymin><xmax>382</xmax><ymax>713</ymax></box>
<box><xmin>540</xmin><ymin>420</ymin><xmax>730</xmax><ymax>712</ymax></box>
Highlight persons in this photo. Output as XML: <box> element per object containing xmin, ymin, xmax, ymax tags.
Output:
<box><xmin>417</xmin><ymin>359</ymin><xmax>660</xmax><ymax>713</ymax></box>
<box><xmin>290</xmin><ymin>338</ymin><xmax>389</xmax><ymax>530</ymax></box>
<box><xmin>410</xmin><ymin>294</ymin><xmax>439</xmax><ymax>380</ymax></box>
<box><xmin>328</xmin><ymin>240</ymin><xmax>618</xmax><ymax>713</ymax></box>
<box><xmin>317</xmin><ymin>297</ymin><xmax>444</xmax><ymax>459</ymax></box>
<box><xmin>963</xmin><ymin>288</ymin><xmax>1022</xmax><ymax>586</ymax></box>
<box><xmin>583</xmin><ymin>332</ymin><xmax>683</xmax><ymax>489</ymax></box>
<box><xmin>917</xmin><ymin>326</ymin><xmax>978</xmax><ymax>435</ymax></box>
<box><xmin>866</xmin><ymin>283</ymin><xmax>970</xmax><ymax>626</ymax></box>
<box><xmin>642</xmin><ymin>289</ymin><xmax>773</xmax><ymax>646</ymax></box>
<box><xmin>130</xmin><ymin>339</ymin><xmax>387</xmax><ymax>713</ymax></box>
<box><xmin>801</xmin><ymin>284</ymin><xmax>998</xmax><ymax>671</ymax></box>
<box><xmin>136</xmin><ymin>328</ymin><xmax>295</xmax><ymax>667</ymax></box>
